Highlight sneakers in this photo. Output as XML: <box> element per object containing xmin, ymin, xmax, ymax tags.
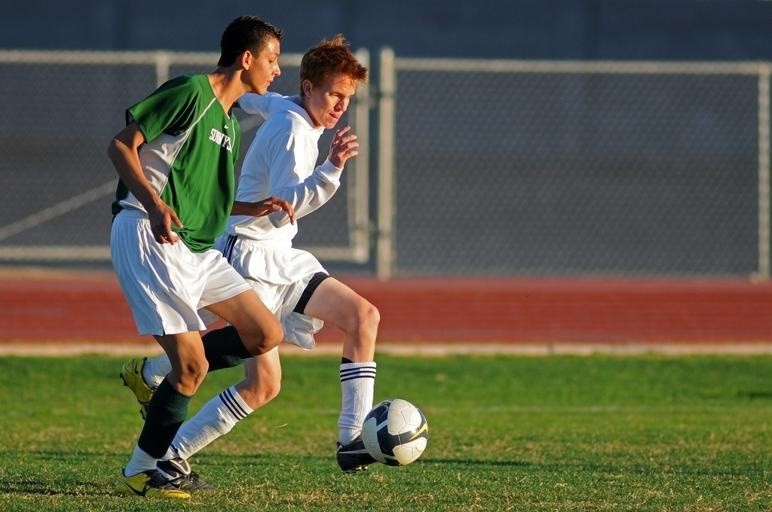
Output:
<box><xmin>156</xmin><ymin>458</ymin><xmax>218</xmax><ymax>494</ymax></box>
<box><xmin>337</xmin><ymin>436</ymin><xmax>378</xmax><ymax>474</ymax></box>
<box><xmin>119</xmin><ymin>357</ymin><xmax>156</xmax><ymax>419</ymax></box>
<box><xmin>116</xmin><ymin>468</ymin><xmax>191</xmax><ymax>500</ymax></box>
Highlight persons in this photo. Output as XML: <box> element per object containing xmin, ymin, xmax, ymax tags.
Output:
<box><xmin>151</xmin><ymin>32</ymin><xmax>387</xmax><ymax>495</ymax></box>
<box><xmin>104</xmin><ymin>12</ymin><xmax>296</xmax><ymax>503</ymax></box>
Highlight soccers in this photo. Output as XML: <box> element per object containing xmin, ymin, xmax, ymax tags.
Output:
<box><xmin>362</xmin><ymin>399</ymin><xmax>429</xmax><ymax>466</ymax></box>
